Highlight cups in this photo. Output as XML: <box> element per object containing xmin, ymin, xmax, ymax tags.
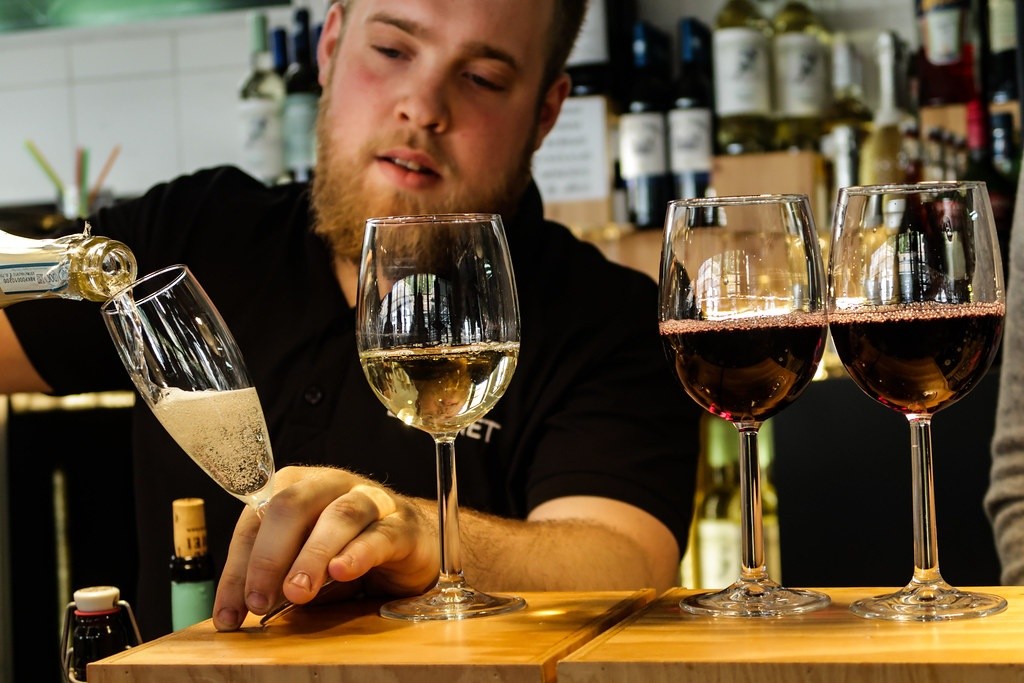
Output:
<box><xmin>58</xmin><ymin>189</ymin><xmax>116</xmax><ymax>221</ymax></box>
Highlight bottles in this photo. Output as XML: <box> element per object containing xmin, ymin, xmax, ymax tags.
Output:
<box><xmin>771</xmin><ymin>0</ymin><xmax>835</xmax><ymax>152</ymax></box>
<box><xmin>286</xmin><ymin>8</ymin><xmax>322</xmax><ymax>182</ymax></box>
<box><xmin>61</xmin><ymin>586</ymin><xmax>142</xmax><ymax>683</ymax></box>
<box><xmin>239</xmin><ymin>14</ymin><xmax>286</xmax><ymax>186</ymax></box>
<box><xmin>819</xmin><ymin>33</ymin><xmax>875</xmax><ymax>227</ymax></box>
<box><xmin>616</xmin><ymin>20</ymin><xmax>671</xmax><ymax>231</ymax></box>
<box><xmin>168</xmin><ymin>498</ymin><xmax>216</xmax><ymax>633</ymax></box>
<box><xmin>981</xmin><ymin>0</ymin><xmax>1024</xmax><ymax>181</ymax></box>
<box><xmin>859</xmin><ymin>29</ymin><xmax>921</xmax><ymax>186</ymax></box>
<box><xmin>712</xmin><ymin>0</ymin><xmax>776</xmax><ymax>153</ymax></box>
<box><xmin>697</xmin><ymin>416</ymin><xmax>781</xmax><ymax>590</ymax></box>
<box><xmin>271</xmin><ymin>28</ymin><xmax>291</xmax><ymax>79</ymax></box>
<box><xmin>909</xmin><ymin>0</ymin><xmax>985</xmax><ymax>182</ymax></box>
<box><xmin>669</xmin><ymin>15</ymin><xmax>719</xmax><ymax>231</ymax></box>
<box><xmin>0</xmin><ymin>221</ymin><xmax>138</xmax><ymax>306</ymax></box>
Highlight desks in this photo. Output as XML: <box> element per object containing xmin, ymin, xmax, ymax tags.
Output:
<box><xmin>87</xmin><ymin>587</ymin><xmax>1024</xmax><ymax>683</ymax></box>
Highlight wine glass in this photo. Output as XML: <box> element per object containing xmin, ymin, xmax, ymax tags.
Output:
<box><xmin>355</xmin><ymin>213</ymin><xmax>528</xmax><ymax>621</ymax></box>
<box><xmin>829</xmin><ymin>181</ymin><xmax>1010</xmax><ymax>623</ymax></box>
<box><xmin>657</xmin><ymin>194</ymin><xmax>829</xmax><ymax>618</ymax></box>
<box><xmin>101</xmin><ymin>264</ymin><xmax>336</xmax><ymax>628</ymax></box>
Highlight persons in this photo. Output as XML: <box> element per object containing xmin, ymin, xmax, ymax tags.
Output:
<box><xmin>981</xmin><ymin>153</ymin><xmax>1024</xmax><ymax>589</ymax></box>
<box><xmin>0</xmin><ymin>0</ymin><xmax>705</xmax><ymax>649</ymax></box>
